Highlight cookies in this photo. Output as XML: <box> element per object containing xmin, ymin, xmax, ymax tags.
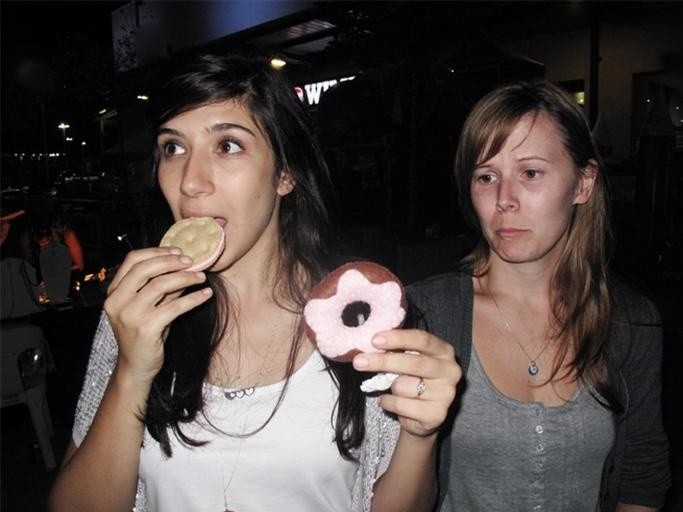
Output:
<box><xmin>159</xmin><ymin>217</ymin><xmax>226</xmax><ymax>274</ymax></box>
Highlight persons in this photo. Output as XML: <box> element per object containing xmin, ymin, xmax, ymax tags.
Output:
<box><xmin>399</xmin><ymin>80</ymin><xmax>673</xmax><ymax>512</ymax></box>
<box><xmin>0</xmin><ymin>205</ymin><xmax>87</xmax><ymax>479</ymax></box>
<box><xmin>47</xmin><ymin>51</ymin><xmax>463</xmax><ymax>512</ymax></box>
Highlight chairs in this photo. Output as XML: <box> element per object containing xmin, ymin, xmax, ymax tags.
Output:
<box><xmin>1</xmin><ymin>323</ymin><xmax>58</xmax><ymax>474</ymax></box>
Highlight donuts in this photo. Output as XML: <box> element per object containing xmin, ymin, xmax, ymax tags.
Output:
<box><xmin>302</xmin><ymin>260</ymin><xmax>409</xmax><ymax>364</ymax></box>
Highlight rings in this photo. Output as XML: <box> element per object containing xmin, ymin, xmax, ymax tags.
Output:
<box><xmin>415</xmin><ymin>378</ymin><xmax>427</xmax><ymax>400</ymax></box>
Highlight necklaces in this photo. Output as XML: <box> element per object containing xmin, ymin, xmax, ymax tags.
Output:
<box><xmin>212</xmin><ymin>301</ymin><xmax>286</xmax><ymax>401</ymax></box>
<box><xmin>482</xmin><ymin>276</ymin><xmax>560</xmax><ymax>376</ymax></box>
